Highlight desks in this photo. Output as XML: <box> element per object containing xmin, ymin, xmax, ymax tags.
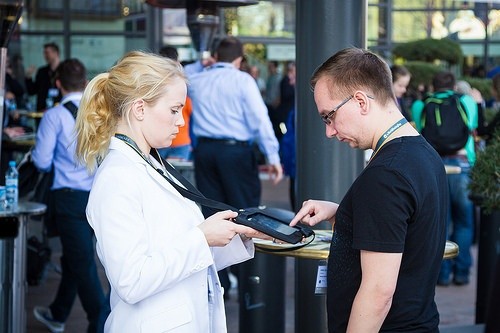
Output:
<box><xmin>0</xmin><ymin>202</ymin><xmax>47</xmax><ymax>333</ymax></box>
<box><xmin>253</xmin><ymin>228</ymin><xmax>459</xmax><ymax>260</ymax></box>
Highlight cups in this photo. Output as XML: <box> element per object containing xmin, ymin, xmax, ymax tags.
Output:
<box><xmin>0</xmin><ymin>186</ymin><xmax>5</xmax><ymax>211</ymax></box>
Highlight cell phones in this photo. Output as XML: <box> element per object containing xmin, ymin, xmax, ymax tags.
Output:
<box><xmin>247</xmin><ymin>213</ymin><xmax>300</xmax><ymax>235</ymax></box>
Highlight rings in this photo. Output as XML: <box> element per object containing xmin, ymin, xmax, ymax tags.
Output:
<box><xmin>272</xmin><ymin>238</ymin><xmax>276</xmax><ymax>243</ymax></box>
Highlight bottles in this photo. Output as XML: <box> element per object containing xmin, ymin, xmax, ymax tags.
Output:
<box><xmin>5</xmin><ymin>161</ymin><xmax>19</xmax><ymax>210</ymax></box>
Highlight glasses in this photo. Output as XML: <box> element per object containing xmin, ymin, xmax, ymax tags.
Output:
<box><xmin>322</xmin><ymin>92</ymin><xmax>375</xmax><ymax>125</ymax></box>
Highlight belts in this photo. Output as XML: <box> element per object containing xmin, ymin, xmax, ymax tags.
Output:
<box><xmin>197</xmin><ymin>136</ymin><xmax>248</xmax><ymax>145</ymax></box>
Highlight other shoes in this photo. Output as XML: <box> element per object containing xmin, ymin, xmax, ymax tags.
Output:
<box><xmin>33</xmin><ymin>304</ymin><xmax>65</xmax><ymax>331</ymax></box>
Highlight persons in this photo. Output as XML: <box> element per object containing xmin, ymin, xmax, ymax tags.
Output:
<box><xmin>453</xmin><ymin>79</ymin><xmax>487</xmax><ymax>137</ymax></box>
<box><xmin>290</xmin><ymin>43</ymin><xmax>448</xmax><ymax>333</ymax></box>
<box><xmin>153</xmin><ymin>47</ymin><xmax>194</xmax><ymax>162</ymax></box>
<box><xmin>0</xmin><ymin>55</ymin><xmax>24</xmax><ymax>187</ymax></box>
<box><xmin>71</xmin><ymin>50</ymin><xmax>304</xmax><ymax>333</ymax></box>
<box><xmin>389</xmin><ymin>64</ymin><xmax>412</xmax><ymax>113</ymax></box>
<box><xmin>181</xmin><ymin>35</ymin><xmax>283</xmax><ymax>302</ymax></box>
<box><xmin>238</xmin><ymin>54</ymin><xmax>297</xmax><ymax>138</ymax></box>
<box><xmin>410</xmin><ymin>71</ymin><xmax>478</xmax><ymax>285</ymax></box>
<box><xmin>469</xmin><ymin>73</ymin><xmax>500</xmax><ymax>146</ymax></box>
<box><xmin>24</xmin><ymin>42</ymin><xmax>61</xmax><ymax>134</ymax></box>
<box><xmin>30</xmin><ymin>58</ymin><xmax>106</xmax><ymax>333</ymax></box>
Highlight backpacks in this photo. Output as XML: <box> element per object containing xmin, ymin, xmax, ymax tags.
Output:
<box><xmin>420</xmin><ymin>90</ymin><xmax>470</xmax><ymax>157</ymax></box>
